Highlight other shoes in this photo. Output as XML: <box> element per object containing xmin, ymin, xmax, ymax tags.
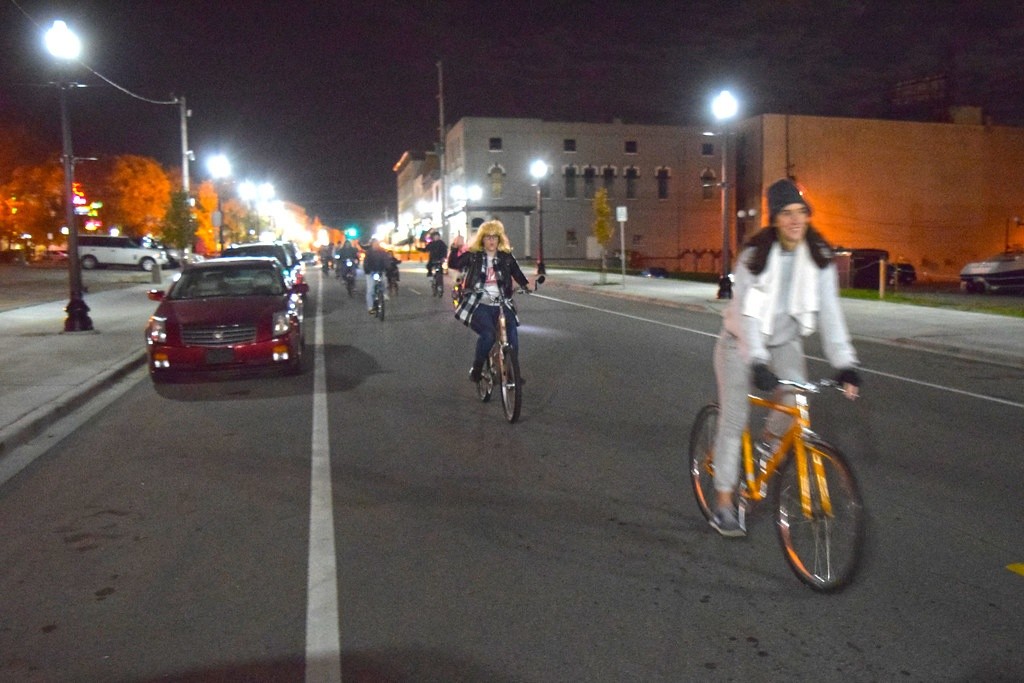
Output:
<box><xmin>708</xmin><ymin>508</ymin><xmax>746</xmax><ymax>536</ymax></box>
<box><xmin>755</xmin><ymin>436</ymin><xmax>775</xmax><ymax>456</ymax></box>
<box><xmin>369</xmin><ymin>309</ymin><xmax>375</xmax><ymax>313</ymax></box>
<box><xmin>468</xmin><ymin>365</ymin><xmax>483</xmax><ymax>381</ymax></box>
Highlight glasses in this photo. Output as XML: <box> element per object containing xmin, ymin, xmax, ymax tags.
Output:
<box><xmin>484</xmin><ymin>234</ymin><xmax>499</xmax><ymax>240</ymax></box>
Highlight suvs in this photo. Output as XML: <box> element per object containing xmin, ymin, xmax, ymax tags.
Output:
<box><xmin>887</xmin><ymin>263</ymin><xmax>916</xmax><ymax>286</ymax></box>
<box><xmin>78</xmin><ymin>235</ymin><xmax>169</xmax><ymax>272</ymax></box>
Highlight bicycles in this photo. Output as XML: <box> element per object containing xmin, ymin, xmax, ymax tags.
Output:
<box><xmin>431</xmin><ymin>259</ymin><xmax>444</xmax><ymax>298</ymax></box>
<box><xmin>452</xmin><ymin>275</ymin><xmax>545</xmax><ymax>424</ymax></box>
<box><xmin>370</xmin><ymin>270</ymin><xmax>385</xmax><ymax>322</ymax></box>
<box><xmin>688</xmin><ymin>379</ymin><xmax>867</xmax><ymax>596</ymax></box>
<box><xmin>387</xmin><ymin>262</ymin><xmax>401</xmax><ymax>293</ymax></box>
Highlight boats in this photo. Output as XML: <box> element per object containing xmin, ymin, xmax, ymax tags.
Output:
<box><xmin>960</xmin><ymin>218</ymin><xmax>1024</xmax><ymax>285</ymax></box>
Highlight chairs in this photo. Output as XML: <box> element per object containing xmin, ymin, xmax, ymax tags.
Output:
<box><xmin>251</xmin><ymin>275</ymin><xmax>279</xmax><ymax>292</ymax></box>
<box><xmin>195</xmin><ymin>277</ymin><xmax>222</xmax><ymax>296</ymax></box>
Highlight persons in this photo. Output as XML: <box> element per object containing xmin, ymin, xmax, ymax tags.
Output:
<box><xmin>416</xmin><ymin>232</ymin><xmax>448</xmax><ymax>277</ymax></box>
<box><xmin>318</xmin><ymin>239</ymin><xmax>402</xmax><ymax>311</ymax></box>
<box><xmin>705</xmin><ymin>178</ymin><xmax>861</xmax><ymax>537</ymax></box>
<box><xmin>448</xmin><ymin>219</ymin><xmax>535</xmax><ymax>386</ymax></box>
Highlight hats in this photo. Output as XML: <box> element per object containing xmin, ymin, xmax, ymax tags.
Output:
<box><xmin>766</xmin><ymin>179</ymin><xmax>807</xmax><ymax>223</ymax></box>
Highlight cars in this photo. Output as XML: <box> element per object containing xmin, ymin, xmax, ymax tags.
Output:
<box><xmin>638</xmin><ymin>267</ymin><xmax>669</xmax><ymax>278</ymax></box>
<box><xmin>132</xmin><ymin>237</ymin><xmax>182</xmax><ymax>270</ymax></box>
<box><xmin>48</xmin><ymin>250</ymin><xmax>68</xmax><ymax>261</ymax></box>
<box><xmin>145</xmin><ymin>241</ymin><xmax>314</xmax><ymax>384</ymax></box>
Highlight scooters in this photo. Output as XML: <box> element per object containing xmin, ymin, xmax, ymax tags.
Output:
<box><xmin>334</xmin><ymin>253</ymin><xmax>359</xmax><ymax>293</ymax></box>
<box><xmin>322</xmin><ymin>257</ymin><xmax>335</xmax><ymax>276</ymax></box>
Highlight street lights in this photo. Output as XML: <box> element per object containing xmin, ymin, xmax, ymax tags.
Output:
<box><xmin>41</xmin><ymin>20</ymin><xmax>101</xmax><ymax>335</ymax></box>
<box><xmin>237</xmin><ymin>180</ymin><xmax>309</xmax><ymax>242</ymax></box>
<box><xmin>702</xmin><ymin>90</ymin><xmax>740</xmax><ymax>300</ymax></box>
<box><xmin>530</xmin><ymin>159</ymin><xmax>549</xmax><ymax>275</ymax></box>
<box><xmin>206</xmin><ymin>153</ymin><xmax>232</xmax><ymax>248</ymax></box>
